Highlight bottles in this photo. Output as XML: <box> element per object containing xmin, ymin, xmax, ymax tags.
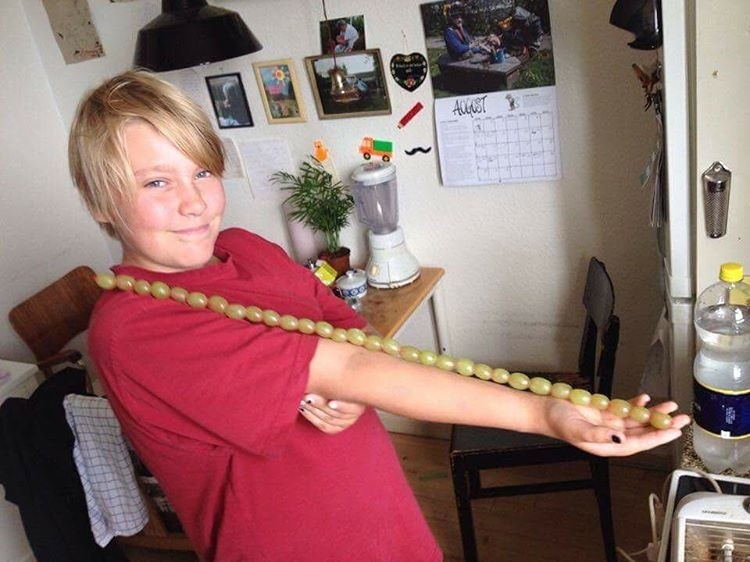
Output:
<box><xmin>692</xmin><ymin>263</ymin><xmax>750</xmax><ymax>475</ymax></box>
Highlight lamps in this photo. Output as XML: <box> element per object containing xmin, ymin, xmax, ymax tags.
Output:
<box><xmin>133</xmin><ymin>0</ymin><xmax>264</xmax><ymax>74</ymax></box>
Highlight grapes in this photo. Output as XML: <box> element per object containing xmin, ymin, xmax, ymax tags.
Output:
<box><xmin>94</xmin><ymin>272</ymin><xmax>675</xmax><ymax>430</ymax></box>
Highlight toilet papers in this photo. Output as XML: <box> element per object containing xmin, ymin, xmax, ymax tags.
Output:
<box><xmin>282</xmin><ymin>197</ymin><xmax>319</xmax><ymax>265</ymax></box>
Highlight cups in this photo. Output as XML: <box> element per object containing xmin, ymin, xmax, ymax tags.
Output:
<box><xmin>336</xmin><ymin>270</ymin><xmax>367</xmax><ymax>299</ymax></box>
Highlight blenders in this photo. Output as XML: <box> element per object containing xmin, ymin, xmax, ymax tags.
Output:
<box><xmin>351</xmin><ymin>162</ymin><xmax>421</xmax><ymax>289</ymax></box>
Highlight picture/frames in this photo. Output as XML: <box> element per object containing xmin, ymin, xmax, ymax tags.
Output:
<box><xmin>204</xmin><ymin>72</ymin><xmax>254</xmax><ymax>129</ymax></box>
<box><xmin>252</xmin><ymin>57</ymin><xmax>308</xmax><ymax>124</ymax></box>
<box><xmin>305</xmin><ymin>48</ymin><xmax>392</xmax><ymax>120</ymax></box>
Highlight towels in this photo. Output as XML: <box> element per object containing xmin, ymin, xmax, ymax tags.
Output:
<box><xmin>62</xmin><ymin>393</ymin><xmax>152</xmax><ymax>550</ymax></box>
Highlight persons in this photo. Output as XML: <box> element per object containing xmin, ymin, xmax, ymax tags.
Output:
<box><xmin>221</xmin><ymin>82</ymin><xmax>249</xmax><ymax>124</ymax></box>
<box><xmin>489</xmin><ymin>0</ymin><xmax>543</xmax><ymax>65</ymax></box>
<box><xmin>444</xmin><ymin>13</ymin><xmax>475</xmax><ymax>60</ymax></box>
<box><xmin>334</xmin><ymin>20</ymin><xmax>358</xmax><ymax>54</ymax></box>
<box><xmin>68</xmin><ymin>71</ymin><xmax>691</xmax><ymax>562</ymax></box>
<box><xmin>345</xmin><ymin>75</ymin><xmax>372</xmax><ymax>105</ymax></box>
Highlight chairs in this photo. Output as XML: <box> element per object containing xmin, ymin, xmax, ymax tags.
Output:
<box><xmin>7</xmin><ymin>266</ymin><xmax>105</xmax><ymax>394</ymax></box>
<box><xmin>449</xmin><ymin>255</ymin><xmax>620</xmax><ymax>562</ymax></box>
<box><xmin>0</xmin><ymin>367</ymin><xmax>199</xmax><ymax>562</ymax></box>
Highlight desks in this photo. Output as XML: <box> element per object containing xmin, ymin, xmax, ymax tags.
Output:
<box><xmin>357</xmin><ymin>266</ymin><xmax>446</xmax><ymax>358</ymax></box>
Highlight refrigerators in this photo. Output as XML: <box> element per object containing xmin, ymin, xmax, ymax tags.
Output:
<box><xmin>662</xmin><ymin>0</ymin><xmax>750</xmax><ymax>416</ymax></box>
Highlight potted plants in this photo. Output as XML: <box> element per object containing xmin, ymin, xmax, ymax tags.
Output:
<box><xmin>267</xmin><ymin>154</ymin><xmax>356</xmax><ymax>280</ymax></box>
